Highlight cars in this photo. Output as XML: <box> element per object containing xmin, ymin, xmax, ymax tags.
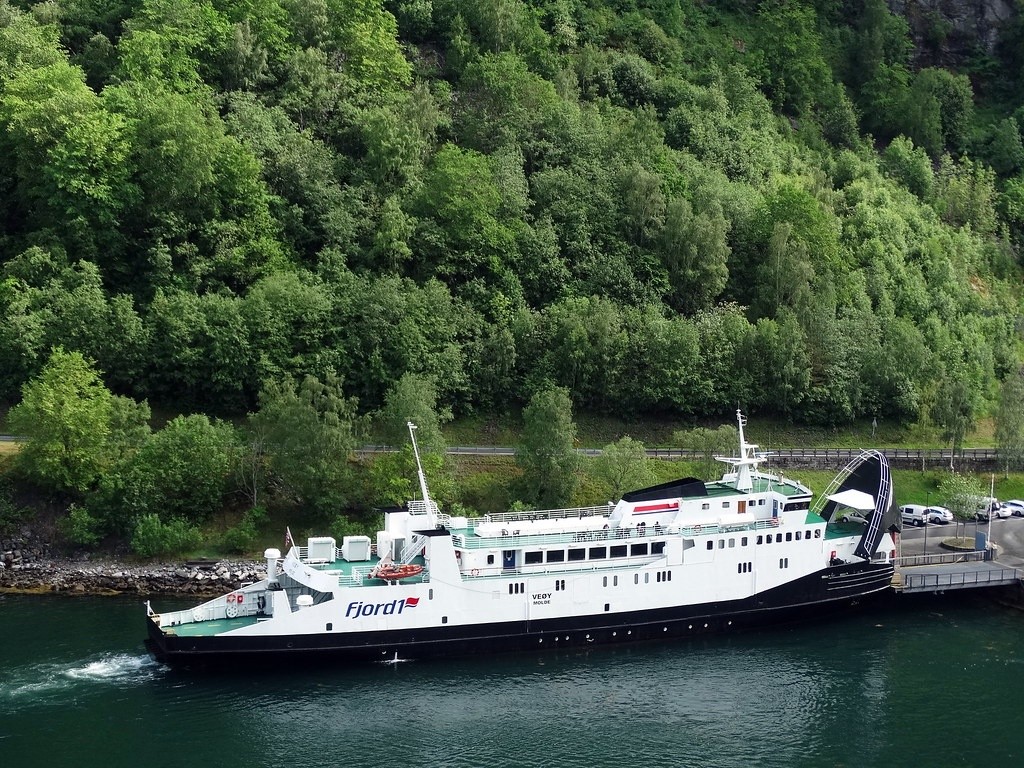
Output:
<box><xmin>842</xmin><ymin>511</ymin><xmax>868</xmax><ymax>526</ymax></box>
<box><xmin>1006</xmin><ymin>499</ymin><xmax>1024</xmax><ymax>518</ymax></box>
<box><xmin>997</xmin><ymin>502</ymin><xmax>1013</xmax><ymax>519</ymax></box>
<box><xmin>927</xmin><ymin>505</ymin><xmax>953</xmax><ymax>524</ymax></box>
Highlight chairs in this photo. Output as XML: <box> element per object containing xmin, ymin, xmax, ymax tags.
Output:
<box><xmin>513</xmin><ymin>530</ymin><xmax>520</xmax><ymax>535</ymax></box>
<box><xmin>502</xmin><ymin>529</ymin><xmax>508</xmax><ymax>535</ymax></box>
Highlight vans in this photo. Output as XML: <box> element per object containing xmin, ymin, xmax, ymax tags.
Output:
<box><xmin>944</xmin><ymin>493</ymin><xmax>999</xmax><ymax>522</ymax></box>
<box><xmin>899</xmin><ymin>504</ymin><xmax>931</xmax><ymax>526</ymax></box>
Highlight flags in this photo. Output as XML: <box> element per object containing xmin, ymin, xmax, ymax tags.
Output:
<box><xmin>285</xmin><ymin>533</ymin><xmax>290</xmax><ymax>547</ymax></box>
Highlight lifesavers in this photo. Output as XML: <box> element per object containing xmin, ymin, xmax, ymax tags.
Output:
<box><xmin>694</xmin><ymin>525</ymin><xmax>702</xmax><ymax>533</ymax></box>
<box><xmin>226</xmin><ymin>594</ymin><xmax>236</xmax><ymax>603</ymax></box>
<box><xmin>236</xmin><ymin>594</ymin><xmax>243</xmax><ymax>603</ymax></box>
<box><xmin>471</xmin><ymin>568</ymin><xmax>479</xmax><ymax>577</ymax></box>
<box><xmin>772</xmin><ymin>517</ymin><xmax>778</xmax><ymax>524</ymax></box>
<box><xmin>256</xmin><ymin>596</ymin><xmax>266</xmax><ymax>608</ymax></box>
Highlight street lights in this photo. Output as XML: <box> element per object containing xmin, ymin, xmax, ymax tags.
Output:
<box><xmin>923</xmin><ymin>491</ymin><xmax>932</xmax><ymax>562</ymax></box>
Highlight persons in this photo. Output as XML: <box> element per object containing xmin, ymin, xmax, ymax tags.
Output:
<box><xmin>603</xmin><ymin>520</ymin><xmax>661</xmax><ymax>540</ymax></box>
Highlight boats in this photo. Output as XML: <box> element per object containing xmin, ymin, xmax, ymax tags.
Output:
<box><xmin>142</xmin><ymin>409</ymin><xmax>903</xmax><ymax>669</ymax></box>
